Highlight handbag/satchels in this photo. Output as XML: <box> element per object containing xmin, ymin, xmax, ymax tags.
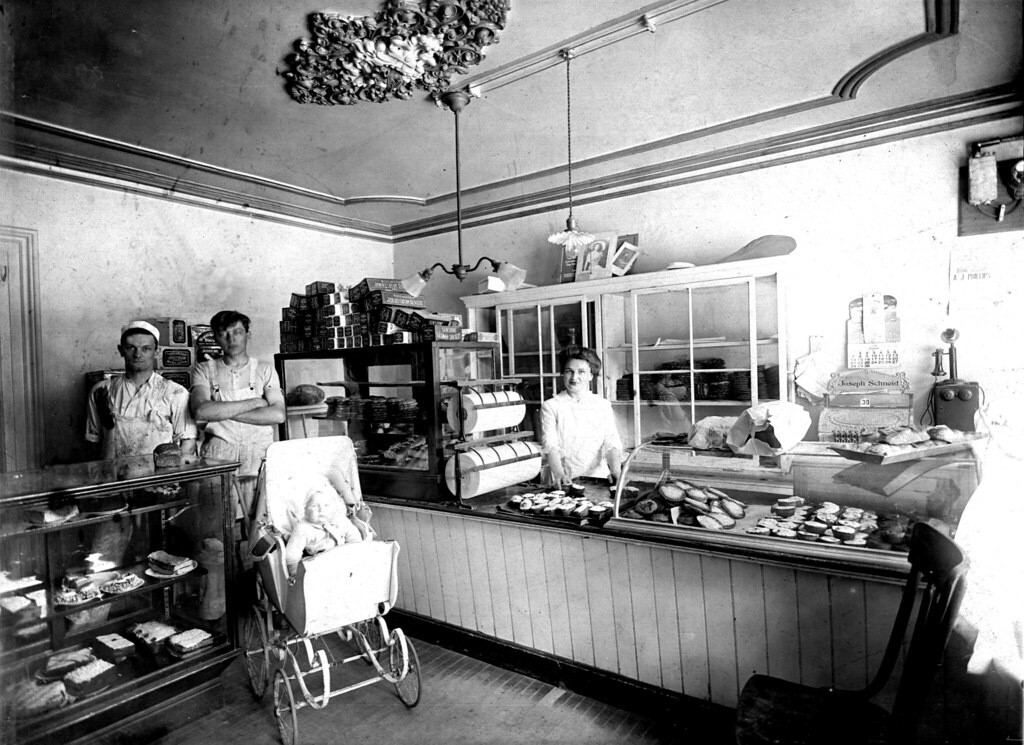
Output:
<box><xmin>726</xmin><ymin>400</ymin><xmax>812</xmax><ymax>456</ymax></box>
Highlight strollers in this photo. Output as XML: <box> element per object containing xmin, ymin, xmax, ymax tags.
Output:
<box><xmin>236</xmin><ymin>434</ymin><xmax>423</xmax><ymax>745</ymax></box>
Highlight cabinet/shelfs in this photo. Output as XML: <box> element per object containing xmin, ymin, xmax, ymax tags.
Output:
<box><xmin>274</xmin><ymin>342</ymin><xmax>502</xmax><ymax>501</ymax></box>
<box><xmin>459</xmin><ymin>255</ymin><xmax>795</xmax><ymax>446</ymax></box>
<box><xmin>0</xmin><ymin>451</ymin><xmax>246</xmax><ymax>745</ymax></box>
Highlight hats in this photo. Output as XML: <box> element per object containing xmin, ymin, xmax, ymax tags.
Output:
<box><xmin>121</xmin><ymin>320</ymin><xmax>160</xmax><ymax>342</ymax></box>
<box><xmin>302</xmin><ymin>487</ymin><xmax>327</xmax><ymax>508</ymax></box>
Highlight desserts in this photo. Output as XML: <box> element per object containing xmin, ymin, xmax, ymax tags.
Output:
<box><xmin>509</xmin><ymin>483</ymin><xmax>639</xmax><ymax>521</ymax></box>
<box><xmin>0</xmin><ymin>481</ymin><xmax>216</xmax><ymax>721</ymax></box>
<box><xmin>745</xmin><ymin>495</ymin><xmax>879</xmax><ymax>548</ymax></box>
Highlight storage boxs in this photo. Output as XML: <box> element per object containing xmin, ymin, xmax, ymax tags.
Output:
<box><xmin>461</xmin><ymin>328</ymin><xmax>474</xmax><ymax>341</ymax></box>
<box><xmin>426</xmin><ymin>313</ymin><xmax>453</xmax><ymax>330</ymax></box>
<box><xmin>478</xmin><ymin>276</ymin><xmax>536</xmax><ymax>295</ymax></box>
<box><xmin>195</xmin><ymin>345</ymin><xmax>223</xmax><ymax>363</ymax></box>
<box><xmin>155</xmin><ymin>370</ymin><xmax>192</xmax><ymax>391</ymax></box>
<box><xmin>154</xmin><ymin>346</ymin><xmax>193</xmax><ymax>370</ymax></box>
<box><xmin>129</xmin><ymin>317</ymin><xmax>188</xmax><ymax>347</ymax></box>
<box><xmin>186</xmin><ymin>324</ymin><xmax>222</xmax><ymax>346</ymax></box>
<box><xmin>423</xmin><ymin>326</ymin><xmax>462</xmax><ymax>342</ymax></box>
<box><xmin>469</xmin><ymin>332</ymin><xmax>499</xmax><ymax>343</ymax></box>
<box><xmin>280</xmin><ymin>278</ymin><xmax>425</xmax><ymax>353</ymax></box>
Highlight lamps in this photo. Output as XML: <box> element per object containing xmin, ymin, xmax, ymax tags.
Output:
<box><xmin>547</xmin><ymin>48</ymin><xmax>595</xmax><ymax>257</ymax></box>
<box><xmin>400</xmin><ymin>90</ymin><xmax>527</xmax><ymax>297</ymax></box>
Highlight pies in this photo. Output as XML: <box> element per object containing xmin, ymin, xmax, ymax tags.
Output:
<box><xmin>324</xmin><ymin>396</ymin><xmax>420</xmax><ymax>421</ymax></box>
<box><xmin>624</xmin><ymin>479</ymin><xmax>745</xmax><ymax>532</ymax></box>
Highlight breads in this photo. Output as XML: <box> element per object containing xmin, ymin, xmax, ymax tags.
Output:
<box><xmin>829</xmin><ymin>424</ymin><xmax>963</xmax><ymax>457</ymax></box>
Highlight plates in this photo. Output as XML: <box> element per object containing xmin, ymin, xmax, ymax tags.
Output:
<box><xmin>35</xmin><ymin>654</ymin><xmax>98</xmax><ymax>677</ymax></box>
<box><xmin>84</xmin><ymin>684</ymin><xmax>111</xmax><ymax>694</ymax></box>
<box><xmin>144</xmin><ymin>560</ymin><xmax>198</xmax><ymax>578</ymax></box>
<box><xmin>54</xmin><ymin>595</ymin><xmax>96</xmax><ymax>606</ymax></box>
<box><xmin>101</xmin><ymin>579</ymin><xmax>144</xmax><ymax>594</ymax></box>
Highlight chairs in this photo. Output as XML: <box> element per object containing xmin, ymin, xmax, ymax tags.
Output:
<box><xmin>727</xmin><ymin>521</ymin><xmax>972</xmax><ymax>745</ymax></box>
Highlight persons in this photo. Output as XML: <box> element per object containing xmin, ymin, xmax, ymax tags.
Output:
<box><xmin>284</xmin><ymin>483</ymin><xmax>363</xmax><ymax>586</ymax></box>
<box><xmin>614</xmin><ymin>250</ymin><xmax>630</xmax><ymax>269</ymax></box>
<box><xmin>583</xmin><ymin>240</ymin><xmax>606</xmax><ymax>278</ymax></box>
<box><xmin>540</xmin><ymin>346</ymin><xmax>630</xmax><ymax>490</ymax></box>
<box><xmin>651</xmin><ymin>372</ymin><xmax>692</xmax><ymax>440</ymax></box>
<box><xmin>189</xmin><ymin>310</ymin><xmax>286</xmax><ymax>634</ymax></box>
<box><xmin>66</xmin><ymin>318</ymin><xmax>198</xmax><ymax>633</ymax></box>
